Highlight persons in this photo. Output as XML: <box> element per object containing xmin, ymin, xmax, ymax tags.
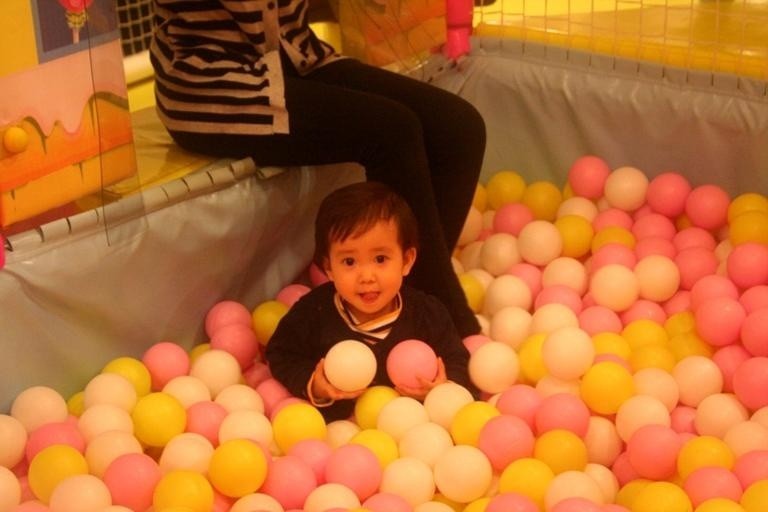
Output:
<box><xmin>147</xmin><ymin>0</ymin><xmax>487</xmax><ymax>340</ymax></box>
<box><xmin>266</xmin><ymin>182</ymin><xmax>480</xmax><ymax>424</ymax></box>
